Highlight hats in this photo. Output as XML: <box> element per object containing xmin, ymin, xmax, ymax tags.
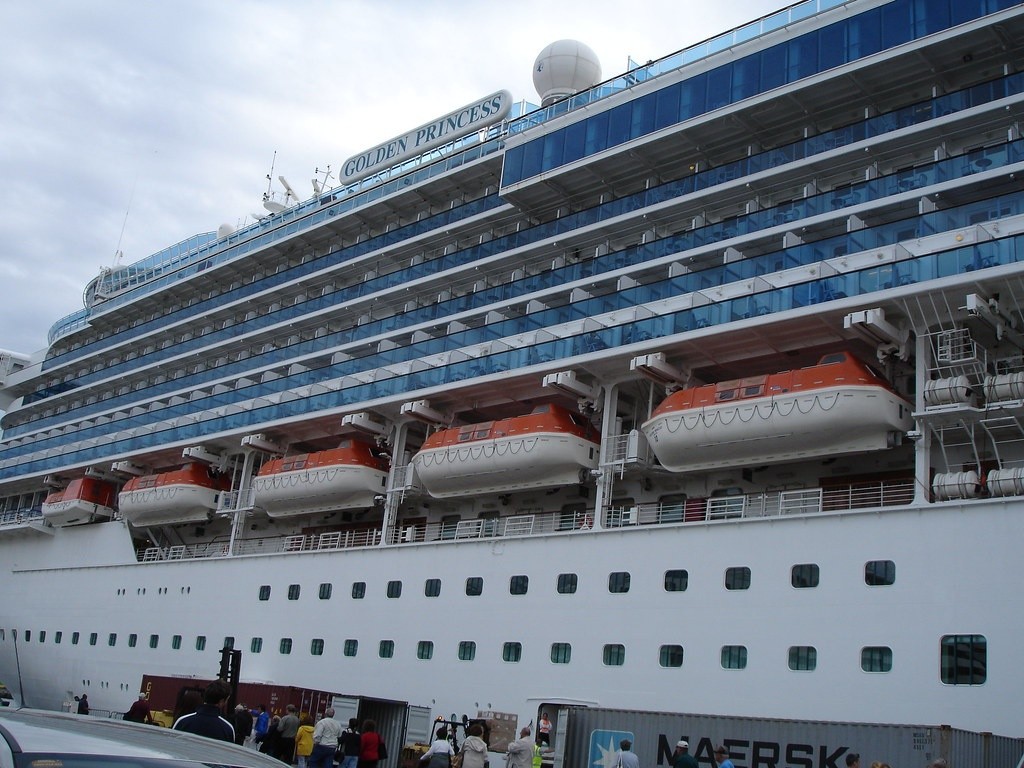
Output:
<box><xmin>677</xmin><ymin>741</ymin><xmax>689</xmax><ymax>749</ymax></box>
<box><xmin>520</xmin><ymin>727</ymin><xmax>530</xmax><ymax>737</ymax></box>
<box><xmin>620</xmin><ymin>739</ymin><xmax>632</xmax><ymax>748</ymax></box>
<box><xmin>139</xmin><ymin>693</ymin><xmax>146</xmax><ymax>698</ymax></box>
<box><xmin>235</xmin><ymin>704</ymin><xmax>244</xmax><ymax>711</ymax></box>
<box><xmin>713</xmin><ymin>745</ymin><xmax>728</xmax><ymax>754</ymax></box>
<box><xmin>436</xmin><ymin>725</ymin><xmax>448</xmax><ymax>740</ymax></box>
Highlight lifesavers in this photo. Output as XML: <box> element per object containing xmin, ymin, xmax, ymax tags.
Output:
<box><xmin>579</xmin><ymin>515</ymin><xmax>593</xmax><ymax>527</ymax></box>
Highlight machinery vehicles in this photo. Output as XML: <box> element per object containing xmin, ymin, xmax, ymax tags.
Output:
<box><xmin>401</xmin><ymin>715</ymin><xmax>510</xmax><ymax>768</ymax></box>
<box><xmin>144</xmin><ymin>644</ymin><xmax>242</xmax><ymax>730</ymax></box>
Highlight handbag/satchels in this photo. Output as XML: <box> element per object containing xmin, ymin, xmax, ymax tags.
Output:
<box><xmin>451</xmin><ymin>753</ymin><xmax>462</xmax><ymax>768</ymax></box>
<box><xmin>377</xmin><ymin>734</ymin><xmax>388</xmax><ymax>760</ymax></box>
<box><xmin>243</xmin><ymin>735</ymin><xmax>263</xmax><ymax>751</ymax></box>
<box><xmin>416</xmin><ymin>759</ymin><xmax>429</xmax><ymax>768</ymax></box>
<box><xmin>333</xmin><ymin>744</ymin><xmax>345</xmax><ymax>763</ymax></box>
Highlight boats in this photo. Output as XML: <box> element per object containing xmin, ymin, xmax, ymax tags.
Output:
<box><xmin>117</xmin><ymin>462</ymin><xmax>231</xmax><ymax>528</ymax></box>
<box><xmin>640</xmin><ymin>352</ymin><xmax>916</xmax><ymax>481</ymax></box>
<box><xmin>412</xmin><ymin>403</ymin><xmax>602</xmax><ymax>504</ymax></box>
<box><xmin>41</xmin><ymin>477</ymin><xmax>118</xmax><ymax>527</ymax></box>
<box><xmin>251</xmin><ymin>438</ymin><xmax>390</xmax><ymax>520</ymax></box>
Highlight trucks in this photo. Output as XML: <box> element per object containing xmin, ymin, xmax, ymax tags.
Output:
<box><xmin>139</xmin><ymin>674</ymin><xmax>432</xmax><ymax>768</ymax></box>
<box><xmin>552</xmin><ymin>706</ymin><xmax>1024</xmax><ymax>768</ymax></box>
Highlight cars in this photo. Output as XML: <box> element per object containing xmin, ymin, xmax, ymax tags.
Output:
<box><xmin>0</xmin><ymin>702</ymin><xmax>293</xmax><ymax>768</ymax></box>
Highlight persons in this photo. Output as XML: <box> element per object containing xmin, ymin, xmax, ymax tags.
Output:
<box><xmin>930</xmin><ymin>760</ymin><xmax>946</xmax><ymax>768</ymax></box>
<box><xmin>309</xmin><ymin>709</ymin><xmax>342</xmax><ymax>768</ymax></box>
<box><xmin>337</xmin><ymin>718</ymin><xmax>361</xmax><ymax>768</ymax></box>
<box><xmin>712</xmin><ymin>746</ymin><xmax>734</xmax><ymax>768</ymax></box>
<box><xmin>274</xmin><ymin>704</ymin><xmax>299</xmax><ymax>766</ymax></box>
<box><xmin>357</xmin><ymin>721</ymin><xmax>385</xmax><ymax>768</ymax></box>
<box><xmin>248</xmin><ymin>704</ymin><xmax>269</xmax><ymax>745</ymax></box>
<box><xmin>532</xmin><ymin>739</ymin><xmax>554</xmax><ymax>768</ymax></box>
<box><xmin>123</xmin><ymin>693</ymin><xmax>154</xmax><ymax>723</ymax></box>
<box><xmin>232</xmin><ymin>704</ymin><xmax>253</xmax><ymax>746</ymax></box>
<box><xmin>673</xmin><ymin>741</ymin><xmax>699</xmax><ymax>768</ymax></box>
<box><xmin>845</xmin><ymin>753</ymin><xmax>891</xmax><ymax>768</ymax></box>
<box><xmin>78</xmin><ymin>694</ymin><xmax>90</xmax><ymax>715</ymax></box>
<box><xmin>169</xmin><ymin>680</ymin><xmax>235</xmax><ymax>744</ymax></box>
<box><xmin>538</xmin><ymin>713</ymin><xmax>553</xmax><ymax>749</ymax></box>
<box><xmin>260</xmin><ymin>716</ymin><xmax>281</xmax><ymax>756</ymax></box>
<box><xmin>610</xmin><ymin>740</ymin><xmax>639</xmax><ymax>768</ymax></box>
<box><xmin>460</xmin><ymin>725</ymin><xmax>488</xmax><ymax>768</ymax></box>
<box><xmin>508</xmin><ymin>727</ymin><xmax>533</xmax><ymax>768</ymax></box>
<box><xmin>295</xmin><ymin>713</ymin><xmax>315</xmax><ymax>768</ymax></box>
<box><xmin>419</xmin><ymin>726</ymin><xmax>456</xmax><ymax>768</ymax></box>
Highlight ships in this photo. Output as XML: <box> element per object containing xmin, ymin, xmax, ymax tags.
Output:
<box><xmin>0</xmin><ymin>1</ymin><xmax>1024</xmax><ymax>768</ymax></box>
